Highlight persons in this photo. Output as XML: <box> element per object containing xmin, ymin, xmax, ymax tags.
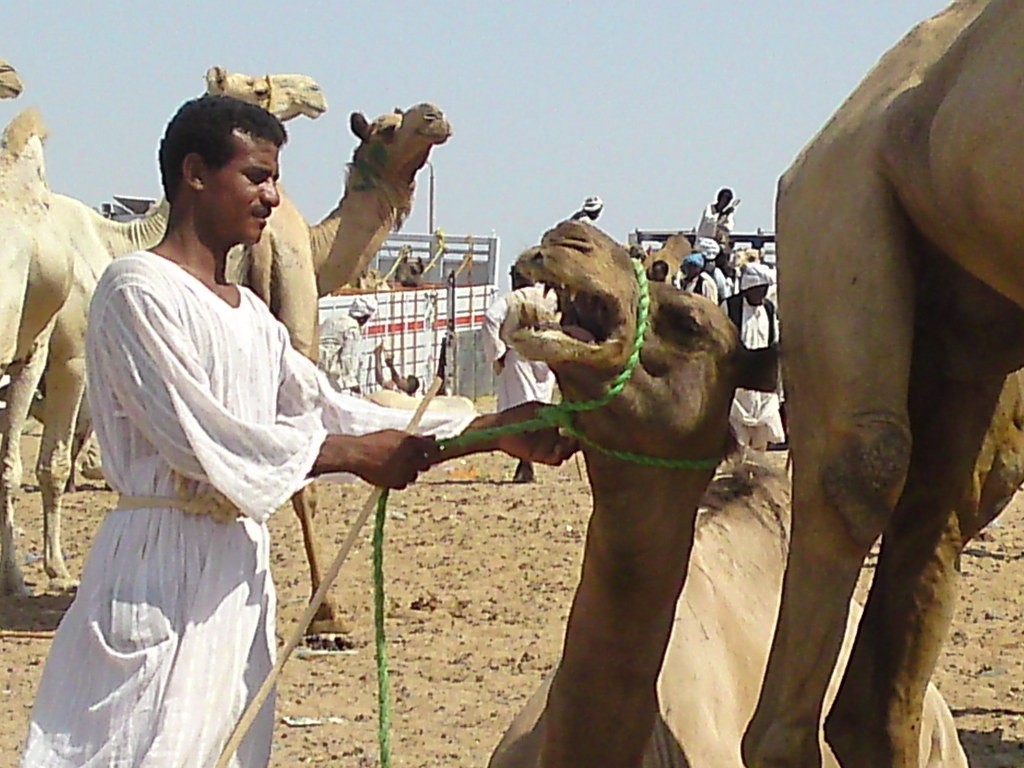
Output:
<box><xmin>316</xmin><ymin>297</ymin><xmax>376</xmax><ymax>394</ymax></box>
<box><xmin>483</xmin><ymin>266</ymin><xmax>556</xmax><ymax>482</ymax></box>
<box><xmin>677</xmin><ymin>236</ymin><xmax>761</xmax><ymax>304</ymax></box>
<box><xmin>18</xmin><ymin>94</ymin><xmax>580</xmax><ymax>768</ymax></box>
<box><xmin>573</xmin><ymin>195</ymin><xmax>604</xmax><ymax>224</ymax></box>
<box><xmin>697</xmin><ymin>188</ymin><xmax>735</xmax><ymax>252</ymax></box>
<box><xmin>374</xmin><ymin>344</ymin><xmax>420</xmax><ymax>398</ymax></box>
<box><xmin>651</xmin><ymin>260</ymin><xmax>669</xmax><ymax>282</ymax></box>
<box><xmin>720</xmin><ymin>263</ymin><xmax>784</xmax><ymax>474</ymax></box>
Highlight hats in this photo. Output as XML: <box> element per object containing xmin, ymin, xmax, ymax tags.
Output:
<box><xmin>699</xmin><ymin>238</ymin><xmax>721</xmax><ymax>259</ymax></box>
<box><xmin>350</xmin><ymin>295</ymin><xmax>378</xmax><ymax>317</ymax></box>
<box><xmin>583</xmin><ymin>195</ymin><xmax>604</xmax><ymax>212</ymax></box>
<box><xmin>684</xmin><ymin>253</ymin><xmax>704</xmax><ymax>267</ymax></box>
<box><xmin>741</xmin><ymin>264</ymin><xmax>773</xmax><ymax>290</ymax></box>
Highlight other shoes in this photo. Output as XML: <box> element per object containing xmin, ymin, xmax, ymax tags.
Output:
<box><xmin>512</xmin><ymin>467</ymin><xmax>535</xmax><ymax>482</ymax></box>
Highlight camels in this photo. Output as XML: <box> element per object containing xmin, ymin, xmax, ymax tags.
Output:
<box><xmin>482</xmin><ymin>1</ymin><xmax>1023</xmax><ymax>767</ymax></box>
<box><xmin>0</xmin><ymin>58</ymin><xmax>457</xmax><ymax>640</ymax></box>
